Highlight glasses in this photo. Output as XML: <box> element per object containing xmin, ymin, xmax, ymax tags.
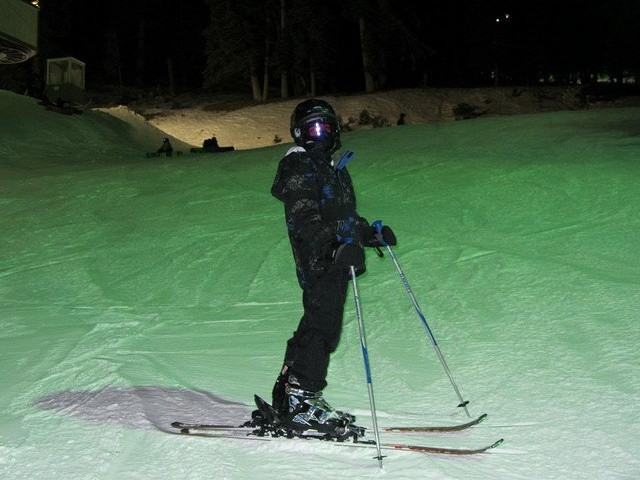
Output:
<box><xmin>296</xmin><ymin>119</ymin><xmax>336</xmax><ymax>138</ymax></box>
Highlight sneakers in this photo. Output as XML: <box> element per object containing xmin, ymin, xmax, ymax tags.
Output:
<box><xmin>273</xmin><ymin>364</ymin><xmax>285</xmax><ymax>410</ymax></box>
<box><xmin>281</xmin><ymin>375</ymin><xmax>350</xmax><ymax>430</ymax></box>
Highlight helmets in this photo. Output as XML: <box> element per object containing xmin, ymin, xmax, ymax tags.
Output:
<box><xmin>289</xmin><ymin>99</ymin><xmax>342</xmax><ymax>151</ymax></box>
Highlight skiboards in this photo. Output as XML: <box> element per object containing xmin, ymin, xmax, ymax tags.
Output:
<box><xmin>171</xmin><ymin>413</ymin><xmax>504</xmax><ymax>455</ymax></box>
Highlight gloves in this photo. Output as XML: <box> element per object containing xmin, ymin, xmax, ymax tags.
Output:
<box><xmin>368</xmin><ymin>220</ymin><xmax>397</xmax><ymax>258</ymax></box>
<box><xmin>326</xmin><ymin>241</ymin><xmax>365</xmax><ymax>267</ymax></box>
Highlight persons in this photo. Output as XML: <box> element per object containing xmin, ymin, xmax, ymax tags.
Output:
<box><xmin>271</xmin><ymin>101</ymin><xmax>396</xmax><ymax>435</ymax></box>
